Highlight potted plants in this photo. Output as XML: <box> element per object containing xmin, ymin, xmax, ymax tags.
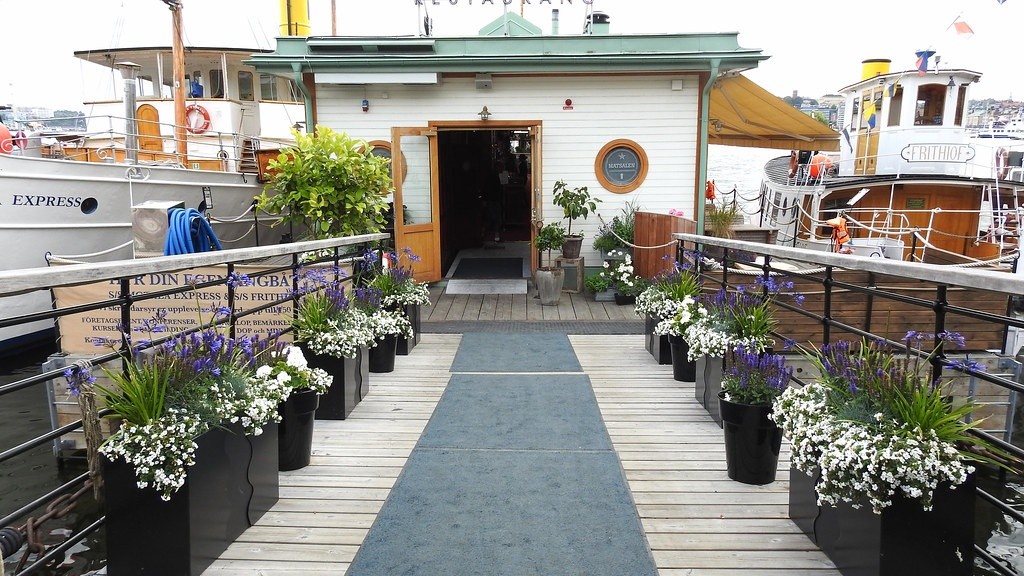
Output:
<box><xmin>552</xmin><ymin>179</ymin><xmax>604</xmax><ymax>259</ymax></box>
<box><xmin>527</xmin><ymin>221</ymin><xmax>568</xmax><ymax>306</ymax></box>
<box><xmin>703</xmin><ymin>194</ymin><xmax>746</xmax><ymax>271</ymax></box>
<box><xmin>592</xmin><ymin>195</ymin><xmax>642</xmax><ymax>275</ymax></box>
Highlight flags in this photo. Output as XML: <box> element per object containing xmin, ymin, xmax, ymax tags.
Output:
<box><xmin>952</xmin><ymin>20</ymin><xmax>973</xmax><ymax>34</ymax></box>
<box><xmin>864</xmin><ymin>102</ymin><xmax>876</xmax><ymax>129</ymax></box>
<box><xmin>915</xmin><ymin>49</ymin><xmax>928</xmax><ymax>77</ymax></box>
<box><xmin>883</xmin><ymin>81</ymin><xmax>898</xmax><ymax>97</ymax></box>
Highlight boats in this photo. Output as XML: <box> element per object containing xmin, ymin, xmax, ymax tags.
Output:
<box><xmin>761</xmin><ymin>58</ymin><xmax>1024</xmax><ymax>265</ymax></box>
<box><xmin>0</xmin><ymin>0</ymin><xmax>311</xmax><ymax>354</ymax></box>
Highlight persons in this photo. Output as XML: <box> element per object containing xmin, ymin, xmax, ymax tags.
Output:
<box><xmin>519</xmin><ymin>154</ymin><xmax>530</xmax><ymax>207</ymax></box>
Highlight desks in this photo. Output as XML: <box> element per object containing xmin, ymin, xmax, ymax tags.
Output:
<box><xmin>554</xmin><ymin>255</ymin><xmax>585</xmax><ymax>294</ymax></box>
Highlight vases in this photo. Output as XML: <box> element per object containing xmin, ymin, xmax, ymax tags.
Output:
<box><xmin>645</xmin><ymin>311</ymin><xmax>673</xmax><ymax>365</ymax></box>
<box><xmin>275</xmin><ymin>387</ymin><xmax>320</xmax><ymax>473</ymax></box>
<box><xmin>593</xmin><ymin>287</ymin><xmax>620</xmax><ymax>302</ymax></box>
<box><xmin>614</xmin><ymin>293</ymin><xmax>642</xmax><ymax>306</ymax></box>
<box><xmin>368</xmin><ymin>331</ymin><xmax>398</xmax><ymax>373</ymax></box>
<box><xmin>717</xmin><ymin>389</ymin><xmax>785</xmax><ymax>486</ymax></box>
<box><xmin>788</xmin><ymin>441</ymin><xmax>976</xmax><ymax>576</ymax></box>
<box><xmin>381</xmin><ymin>300</ymin><xmax>421</xmax><ymax>356</ymax></box>
<box><xmin>695</xmin><ymin>346</ymin><xmax>774</xmax><ymax>429</ymax></box>
<box><xmin>294</xmin><ymin>342</ymin><xmax>369</xmax><ymax>421</ymax></box>
<box><xmin>668</xmin><ymin>334</ymin><xmax>696</xmax><ymax>382</ymax></box>
<box><xmin>102</xmin><ymin>408</ymin><xmax>279</xmax><ymax>576</ymax></box>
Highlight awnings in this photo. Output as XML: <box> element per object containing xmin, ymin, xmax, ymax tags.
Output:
<box><xmin>707</xmin><ymin>70</ymin><xmax>843</xmax><ymax>153</ymax></box>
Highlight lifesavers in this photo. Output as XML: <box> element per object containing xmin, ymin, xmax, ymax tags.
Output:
<box><xmin>995</xmin><ymin>146</ymin><xmax>1009</xmax><ymax>181</ymax></box>
<box><xmin>185</xmin><ymin>104</ymin><xmax>210</xmax><ymax>134</ymax></box>
<box><xmin>788</xmin><ymin>150</ymin><xmax>800</xmax><ymax>178</ymax></box>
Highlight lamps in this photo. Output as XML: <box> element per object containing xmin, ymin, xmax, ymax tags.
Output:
<box><xmin>477</xmin><ymin>106</ymin><xmax>493</xmax><ymax>121</ymax></box>
<box><xmin>197</xmin><ymin>186</ymin><xmax>213</xmax><ymax>216</ymax></box>
<box><xmin>292</xmin><ymin>121</ymin><xmax>306</xmax><ymax>133</ymax></box>
<box><xmin>475</xmin><ymin>73</ymin><xmax>492</xmax><ymax>91</ymax></box>
<box><xmin>314</xmin><ymin>73</ymin><xmax>443</xmax><ymax>87</ymax></box>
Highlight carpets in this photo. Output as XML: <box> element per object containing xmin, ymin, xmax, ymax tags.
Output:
<box><xmin>341</xmin><ymin>449</ymin><xmax>661</xmax><ymax>576</ymax></box>
<box><xmin>451</xmin><ymin>257</ymin><xmax>523</xmax><ymax>278</ymax></box>
<box><xmin>413</xmin><ymin>374</ymin><xmax>615</xmax><ymax>451</ymax></box>
<box><xmin>449</xmin><ymin>331</ymin><xmax>585</xmax><ymax>374</ymax></box>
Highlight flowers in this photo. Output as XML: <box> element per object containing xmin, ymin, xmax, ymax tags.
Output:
<box><xmin>682</xmin><ymin>273</ymin><xmax>805</xmax><ymax>362</ymax></box>
<box><xmin>353</xmin><ymin>287</ymin><xmax>415</xmax><ymax>340</ymax></box>
<box><xmin>64</xmin><ymin>271</ymin><xmax>336</xmax><ymax>502</ymax></box>
<box><xmin>584</xmin><ymin>249</ymin><xmax>651</xmax><ymax>298</ymax></box>
<box><xmin>720</xmin><ymin>340</ymin><xmax>794</xmax><ymax>404</ymax></box>
<box><xmin>633</xmin><ymin>250</ymin><xmax>708</xmax><ymax>321</ymax></box>
<box><xmin>273</xmin><ymin>265</ymin><xmax>379</xmax><ymax>359</ymax></box>
<box><xmin>651</xmin><ymin>295</ymin><xmax>712</xmax><ymax>336</ymax></box>
<box><xmin>362</xmin><ymin>247</ymin><xmax>432</xmax><ymax>309</ymax></box>
<box><xmin>766</xmin><ymin>329</ymin><xmax>1017</xmax><ymax>515</ymax></box>
